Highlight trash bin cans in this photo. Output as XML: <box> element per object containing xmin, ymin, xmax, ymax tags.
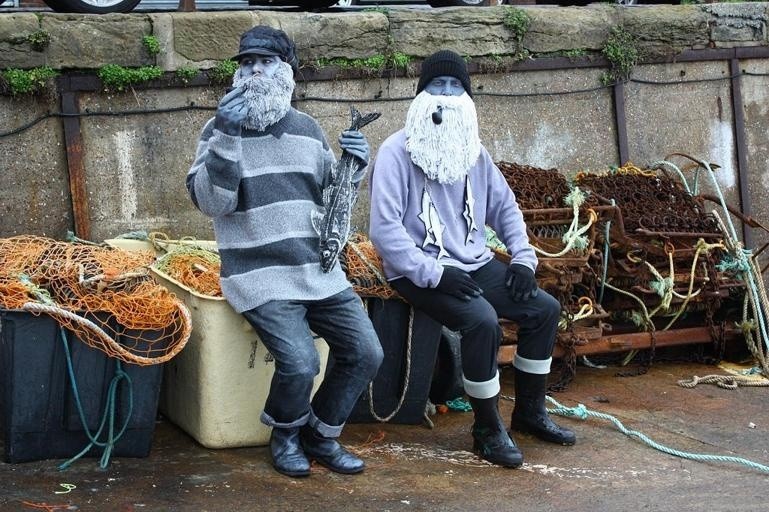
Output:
<box><xmin>313</xmin><ymin>238</ymin><xmax>444</xmax><ymax>426</ymax></box>
<box><xmin>107</xmin><ymin>238</ymin><xmax>331</xmax><ymax>451</ymax></box>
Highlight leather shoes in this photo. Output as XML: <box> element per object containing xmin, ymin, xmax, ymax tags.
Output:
<box><xmin>303</xmin><ymin>432</ymin><xmax>365</xmax><ymax>474</ymax></box>
<box><xmin>268</xmin><ymin>428</ymin><xmax>311</xmax><ymax>478</ymax></box>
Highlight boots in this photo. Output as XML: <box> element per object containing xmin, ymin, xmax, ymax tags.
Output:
<box><xmin>510</xmin><ymin>350</ymin><xmax>576</xmax><ymax>445</ymax></box>
<box><xmin>460</xmin><ymin>369</ymin><xmax>525</xmax><ymax>468</ymax></box>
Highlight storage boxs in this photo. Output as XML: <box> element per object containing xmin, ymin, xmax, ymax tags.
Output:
<box><xmin>103</xmin><ymin>237</ymin><xmax>440</xmax><ymax>449</ymax></box>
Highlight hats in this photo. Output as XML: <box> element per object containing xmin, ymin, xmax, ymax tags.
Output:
<box><xmin>228</xmin><ymin>23</ymin><xmax>302</xmax><ymax>82</ymax></box>
<box><xmin>415</xmin><ymin>48</ymin><xmax>475</xmax><ymax>102</ymax></box>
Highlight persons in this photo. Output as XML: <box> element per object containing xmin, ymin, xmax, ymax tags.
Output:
<box><xmin>184</xmin><ymin>25</ymin><xmax>386</xmax><ymax>477</ymax></box>
<box><xmin>367</xmin><ymin>48</ymin><xmax>576</xmax><ymax>466</ymax></box>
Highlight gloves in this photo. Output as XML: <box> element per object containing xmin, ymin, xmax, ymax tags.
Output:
<box><xmin>213</xmin><ymin>85</ymin><xmax>251</xmax><ymax>140</ymax></box>
<box><xmin>503</xmin><ymin>262</ymin><xmax>538</xmax><ymax>302</ymax></box>
<box><xmin>437</xmin><ymin>264</ymin><xmax>486</xmax><ymax>301</ymax></box>
<box><xmin>338</xmin><ymin>127</ymin><xmax>372</xmax><ymax>175</ymax></box>
